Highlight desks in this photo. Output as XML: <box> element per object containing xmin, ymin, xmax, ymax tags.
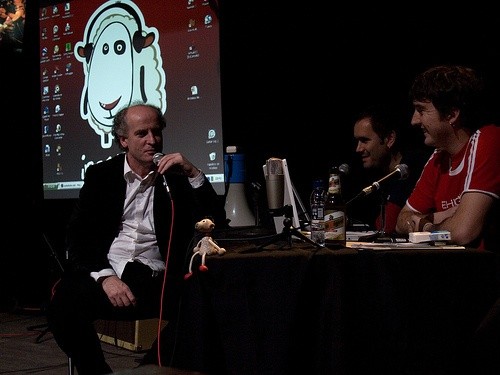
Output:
<box><xmin>169</xmin><ymin>244</ymin><xmax>500</xmax><ymax>375</ymax></box>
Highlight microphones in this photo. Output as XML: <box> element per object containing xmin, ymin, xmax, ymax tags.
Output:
<box><xmin>363</xmin><ymin>164</ymin><xmax>409</xmax><ymax>197</ymax></box>
<box><xmin>337</xmin><ymin>163</ymin><xmax>350</xmax><ymax>180</ymax></box>
<box><xmin>154</xmin><ymin>152</ymin><xmax>170</xmax><ymax>194</ymax></box>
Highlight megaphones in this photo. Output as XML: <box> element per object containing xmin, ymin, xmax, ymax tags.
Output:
<box><xmin>223</xmin><ymin>153</ymin><xmax>258</xmax><ymax>227</ymax></box>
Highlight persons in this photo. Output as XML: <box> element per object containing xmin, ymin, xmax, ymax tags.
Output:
<box><xmin>43</xmin><ymin>105</ymin><xmax>228</xmax><ymax>375</ymax></box>
<box><xmin>346</xmin><ymin>112</ymin><xmax>419</xmax><ymax>225</ymax></box>
<box><xmin>396</xmin><ymin>66</ymin><xmax>500</xmax><ymax>246</ymax></box>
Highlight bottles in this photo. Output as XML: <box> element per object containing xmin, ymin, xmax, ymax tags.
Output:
<box><xmin>322</xmin><ymin>167</ymin><xmax>346</xmax><ymax>250</ymax></box>
<box><xmin>310</xmin><ymin>179</ymin><xmax>327</xmax><ymax>247</ymax></box>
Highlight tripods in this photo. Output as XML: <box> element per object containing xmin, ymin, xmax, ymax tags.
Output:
<box><xmin>237</xmin><ymin>204</ymin><xmax>324</xmax><ymax>253</ymax></box>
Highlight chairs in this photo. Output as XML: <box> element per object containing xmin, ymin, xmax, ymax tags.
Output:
<box><xmin>41</xmin><ymin>231</ymin><xmax>76</xmax><ymax>375</ymax></box>
